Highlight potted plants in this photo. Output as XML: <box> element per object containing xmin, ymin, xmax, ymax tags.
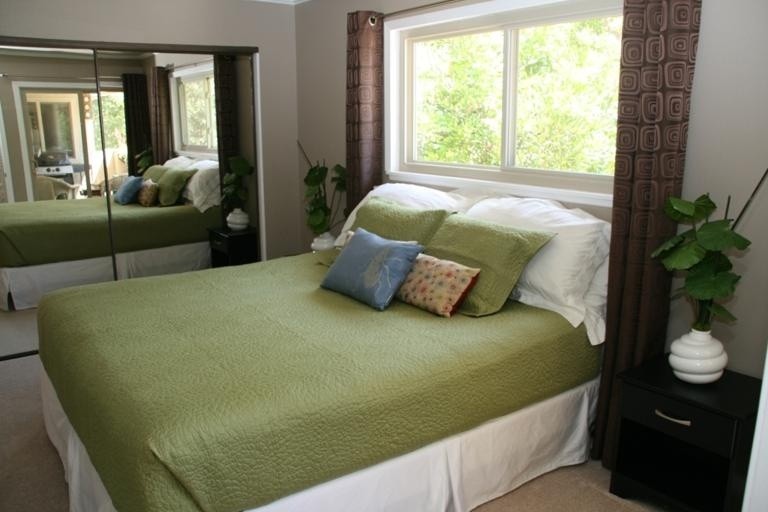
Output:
<box><xmin>650</xmin><ymin>192</ymin><xmax>752</xmax><ymax>386</ymax></box>
<box><xmin>221</xmin><ymin>156</ymin><xmax>255</xmax><ymax>231</ymax></box>
<box><xmin>302</xmin><ymin>160</ymin><xmax>358</xmax><ymax>252</ymax></box>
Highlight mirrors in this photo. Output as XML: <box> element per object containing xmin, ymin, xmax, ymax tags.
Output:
<box><xmin>0</xmin><ymin>35</ymin><xmax>266</xmax><ymax>361</ymax></box>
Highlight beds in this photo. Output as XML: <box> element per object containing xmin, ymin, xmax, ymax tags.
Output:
<box><xmin>37</xmin><ymin>248</ymin><xmax>607</xmax><ymax>512</ymax></box>
<box><xmin>0</xmin><ymin>197</ymin><xmax>222</xmax><ymax>312</ymax></box>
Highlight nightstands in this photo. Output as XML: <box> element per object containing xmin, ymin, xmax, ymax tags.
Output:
<box><xmin>609</xmin><ymin>354</ymin><xmax>762</xmax><ymax>512</ymax></box>
<box><xmin>205</xmin><ymin>228</ymin><xmax>259</xmax><ymax>268</ymax></box>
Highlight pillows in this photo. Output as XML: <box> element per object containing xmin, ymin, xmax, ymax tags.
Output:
<box><xmin>463</xmin><ymin>195</ymin><xmax>612</xmax><ymax>329</ymax></box>
<box><xmin>113</xmin><ymin>156</ymin><xmax>222</xmax><ymax>214</ymax></box>
<box><xmin>423</xmin><ymin>213</ymin><xmax>559</xmax><ymax>317</ymax></box>
<box><xmin>320</xmin><ymin>226</ymin><xmax>425</xmax><ymax>311</ymax></box>
<box><xmin>349</xmin><ymin>195</ymin><xmax>459</xmax><ymax>246</ymax></box>
<box><xmin>369</xmin><ymin>182</ymin><xmax>488</xmax><ymax>213</ymax></box>
<box><xmin>396</xmin><ymin>253</ymin><xmax>482</xmax><ymax>318</ymax></box>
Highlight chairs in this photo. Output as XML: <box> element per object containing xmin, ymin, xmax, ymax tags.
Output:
<box><xmin>33</xmin><ymin>175</ymin><xmax>81</xmax><ymax>200</ymax></box>
